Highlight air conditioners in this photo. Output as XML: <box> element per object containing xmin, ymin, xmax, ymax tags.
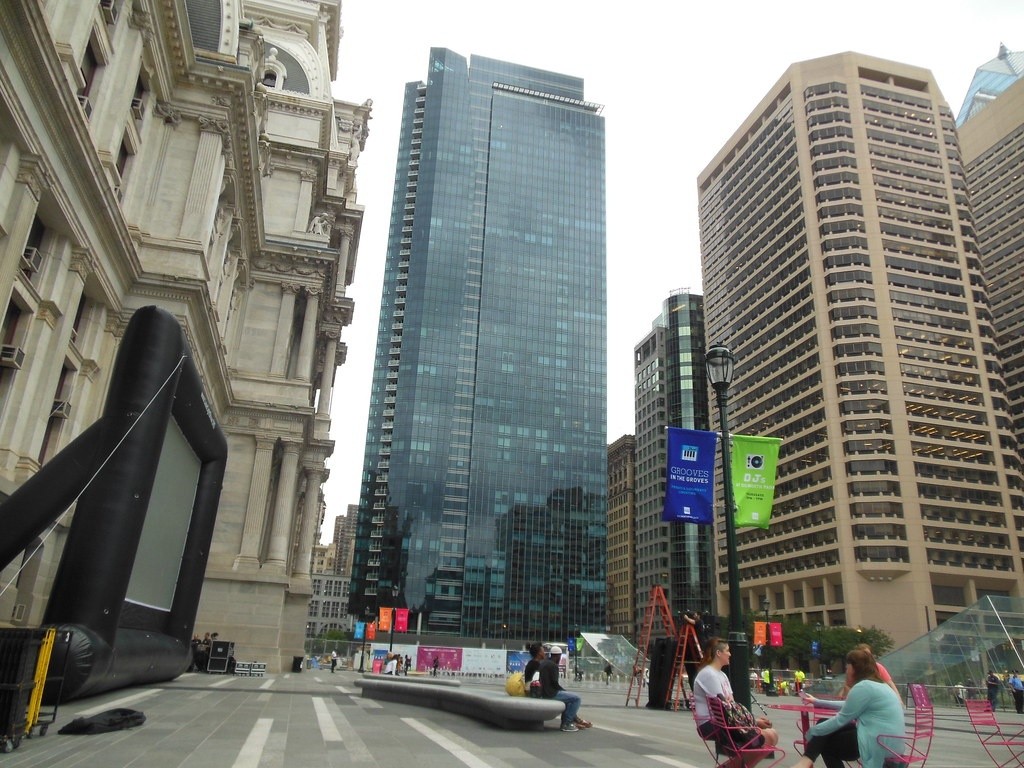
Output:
<box><xmin>101</xmin><ymin>0</ymin><xmax>119</xmax><ymax>24</ymax></box>
<box><xmin>0</xmin><ymin>344</ymin><xmax>25</xmax><ymax>370</ymax></box>
<box><xmin>132</xmin><ymin>98</ymin><xmax>145</xmax><ymax>120</ymax></box>
<box><xmin>25</xmin><ymin>246</ymin><xmax>44</xmax><ymax>273</ymax></box>
<box><xmin>51</xmin><ymin>399</ymin><xmax>72</xmax><ymax>418</ymax></box>
<box><xmin>78</xmin><ymin>95</ymin><xmax>93</xmax><ymax>122</ymax></box>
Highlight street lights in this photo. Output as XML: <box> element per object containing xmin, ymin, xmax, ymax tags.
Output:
<box><xmin>358</xmin><ymin>606</ymin><xmax>370</xmax><ymax>672</ymax></box>
<box><xmin>704</xmin><ymin>340</ymin><xmax>751</xmax><ymax>709</ymax></box>
<box><xmin>816</xmin><ymin>622</ymin><xmax>825</xmax><ymax>678</ymax></box>
<box><xmin>763</xmin><ymin>599</ymin><xmax>776</xmax><ymax>696</ymax></box>
<box><xmin>390</xmin><ymin>584</ymin><xmax>398</xmax><ymax>652</ymax></box>
<box><xmin>574</xmin><ymin>624</ymin><xmax>579</xmax><ymax>678</ymax></box>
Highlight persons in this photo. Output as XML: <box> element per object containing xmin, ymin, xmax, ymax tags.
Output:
<box><xmin>694</xmin><ymin>638</ymin><xmax>778</xmax><ymax>768</ymax></box>
<box><xmin>604</xmin><ymin>663</ymin><xmax>612</xmax><ymax>685</ymax></box>
<box><xmin>683</xmin><ymin>611</ymin><xmax>707</xmax><ymax>658</ymax></box>
<box><xmin>191</xmin><ymin>632</ymin><xmax>219</xmax><ymax>671</ymax></box>
<box><xmin>525</xmin><ymin>642</ymin><xmax>593</xmax><ymax>728</ymax></box>
<box><xmin>966</xmin><ymin>678</ymin><xmax>976</xmax><ymax>699</ymax></box>
<box><xmin>433</xmin><ymin>656</ymin><xmax>438</xmax><ymax>676</ymax></box>
<box><xmin>986</xmin><ymin>670</ymin><xmax>1001</xmax><ymax>712</ymax></box>
<box><xmin>792</xmin><ymin>649</ymin><xmax>905</xmax><ymax>768</ymax></box>
<box><xmin>331</xmin><ymin>649</ymin><xmax>337</xmax><ymax>673</ymax></box>
<box><xmin>794</xmin><ymin>666</ymin><xmax>805</xmax><ymax>693</ymax></box>
<box><xmin>540</xmin><ymin>647</ymin><xmax>581</xmax><ymax>732</ymax></box>
<box><xmin>383</xmin><ymin>652</ymin><xmax>408</xmax><ymax>676</ymax></box>
<box><xmin>1009</xmin><ymin>669</ymin><xmax>1024</xmax><ymax>713</ymax></box>
<box><xmin>750</xmin><ymin>667</ymin><xmax>791</xmax><ymax>695</ymax></box>
<box><xmin>847</xmin><ymin>644</ymin><xmax>905</xmax><ymax>709</ymax></box>
<box><xmin>954</xmin><ymin>682</ymin><xmax>966</xmax><ymax>707</ymax></box>
<box><xmin>637</xmin><ymin>667</ymin><xmax>649</xmax><ymax>687</ymax></box>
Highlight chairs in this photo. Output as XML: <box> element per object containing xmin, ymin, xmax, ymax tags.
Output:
<box><xmin>963</xmin><ymin>699</ymin><xmax>1024</xmax><ymax>768</ymax></box>
<box><xmin>688</xmin><ymin>692</ymin><xmax>785</xmax><ymax>768</ymax></box>
<box><xmin>877</xmin><ymin>683</ymin><xmax>934</xmax><ymax>768</ymax></box>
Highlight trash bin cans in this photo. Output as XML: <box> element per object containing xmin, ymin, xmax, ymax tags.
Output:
<box><xmin>292</xmin><ymin>657</ymin><xmax>303</xmax><ymax>672</ymax></box>
<box><xmin>307</xmin><ymin>660</ymin><xmax>312</xmax><ymax>669</ymax></box>
<box><xmin>0</xmin><ymin>627</ymin><xmax>48</xmax><ymax>753</ymax></box>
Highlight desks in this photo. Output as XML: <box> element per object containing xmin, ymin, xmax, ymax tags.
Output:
<box><xmin>769</xmin><ymin>705</ymin><xmax>856</xmax><ymax>768</ymax></box>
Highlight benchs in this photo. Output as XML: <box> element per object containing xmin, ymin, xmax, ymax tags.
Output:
<box><xmin>366</xmin><ymin>672</ymin><xmax>462</xmax><ymax>686</ymax></box>
<box><xmin>355</xmin><ymin>678</ymin><xmax>566</xmax><ymax>729</ymax></box>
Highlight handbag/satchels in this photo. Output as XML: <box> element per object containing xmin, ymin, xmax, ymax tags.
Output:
<box><xmin>718</xmin><ymin>693</ymin><xmax>766</xmax><ymax>749</ymax></box>
<box><xmin>505</xmin><ymin>671</ymin><xmax>526</xmax><ymax>697</ymax></box>
<box><xmin>530</xmin><ymin>681</ymin><xmax>543</xmax><ymax>698</ymax></box>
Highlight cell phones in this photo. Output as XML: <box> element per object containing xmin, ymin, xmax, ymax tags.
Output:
<box><xmin>799</xmin><ymin>689</ymin><xmax>808</xmax><ymax>700</ymax></box>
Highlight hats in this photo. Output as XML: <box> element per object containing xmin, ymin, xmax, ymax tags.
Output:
<box><xmin>1012</xmin><ymin>669</ymin><xmax>1019</xmax><ymax>673</ymax></box>
<box><xmin>550</xmin><ymin>645</ymin><xmax>563</xmax><ymax>654</ymax></box>
<box><xmin>987</xmin><ymin>670</ymin><xmax>993</xmax><ymax>674</ymax></box>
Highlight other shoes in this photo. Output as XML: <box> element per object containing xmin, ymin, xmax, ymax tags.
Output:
<box><xmin>576</xmin><ymin>724</ymin><xmax>586</xmax><ymax>730</ymax></box>
<box><xmin>563</xmin><ymin>725</ymin><xmax>579</xmax><ymax>732</ymax></box>
<box><xmin>560</xmin><ymin>725</ymin><xmax>567</xmax><ymax>731</ymax></box>
<box><xmin>581</xmin><ymin>720</ymin><xmax>593</xmax><ymax>727</ymax></box>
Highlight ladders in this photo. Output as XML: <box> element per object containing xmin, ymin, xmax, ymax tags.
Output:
<box><xmin>664</xmin><ymin>622</ymin><xmax>704</xmax><ymax>712</ymax></box>
<box><xmin>625</xmin><ymin>584</ymin><xmax>687</xmax><ymax>708</ymax></box>
